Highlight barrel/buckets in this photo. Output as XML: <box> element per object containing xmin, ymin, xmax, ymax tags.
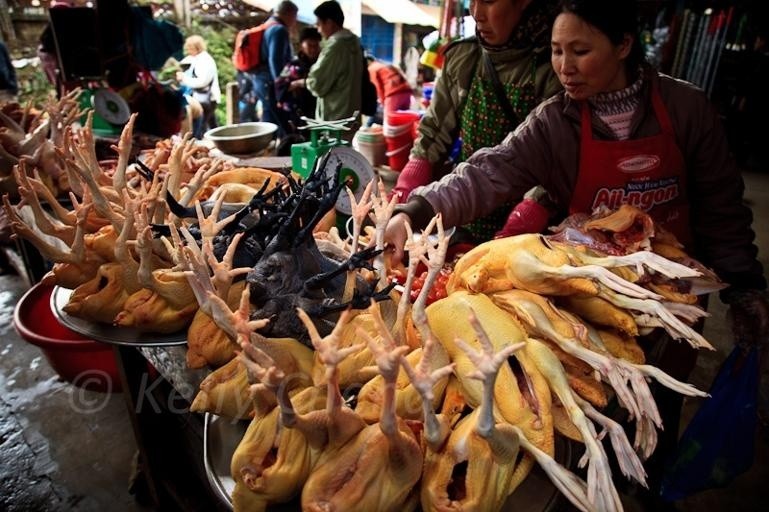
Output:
<box><xmin>359</xmin><ymin>111</ymin><xmax>421</xmax><ymax>172</ymax></box>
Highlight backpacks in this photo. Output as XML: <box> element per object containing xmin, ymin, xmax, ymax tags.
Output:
<box><xmin>233</xmin><ymin>22</ymin><xmax>278</xmax><ymax>72</ymax></box>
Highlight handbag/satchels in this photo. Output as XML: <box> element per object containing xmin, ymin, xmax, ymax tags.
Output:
<box><xmin>193</xmin><ymin>91</ymin><xmax>211</xmax><ymax>109</ymax></box>
<box><xmin>361</xmin><ymin>72</ymin><xmax>377</xmax><ymax>117</ymax></box>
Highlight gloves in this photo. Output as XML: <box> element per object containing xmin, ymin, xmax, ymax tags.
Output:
<box><xmin>493</xmin><ymin>199</ymin><xmax>548</xmax><ymax>240</ymax></box>
<box><xmin>388</xmin><ymin>159</ymin><xmax>431</xmax><ymax>205</ymax></box>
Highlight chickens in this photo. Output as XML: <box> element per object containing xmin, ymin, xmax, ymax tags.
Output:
<box><xmin>10</xmin><ymin>84</ymin><xmax>528</xmax><ymax>512</ymax></box>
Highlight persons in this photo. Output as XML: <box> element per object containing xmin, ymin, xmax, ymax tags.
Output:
<box><xmin>385</xmin><ymin>0</ymin><xmax>568</xmax><ymax>238</ymax></box>
<box><xmin>363</xmin><ymin>0</ymin><xmax>768</xmax><ymax>511</ymax></box>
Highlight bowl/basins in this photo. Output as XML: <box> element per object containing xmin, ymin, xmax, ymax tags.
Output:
<box><xmin>12</xmin><ymin>275</ymin><xmax>130</xmax><ymax>395</ymax></box>
<box><xmin>204</xmin><ymin>121</ymin><xmax>280</xmax><ymax>155</ymax></box>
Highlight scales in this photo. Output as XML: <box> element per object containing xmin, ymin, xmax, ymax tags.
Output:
<box><xmin>70</xmin><ymin>70</ymin><xmax>131</xmax><ymax>136</ymax></box>
<box><xmin>290</xmin><ymin>116</ymin><xmax>379</xmax><ymax>239</ymax></box>
